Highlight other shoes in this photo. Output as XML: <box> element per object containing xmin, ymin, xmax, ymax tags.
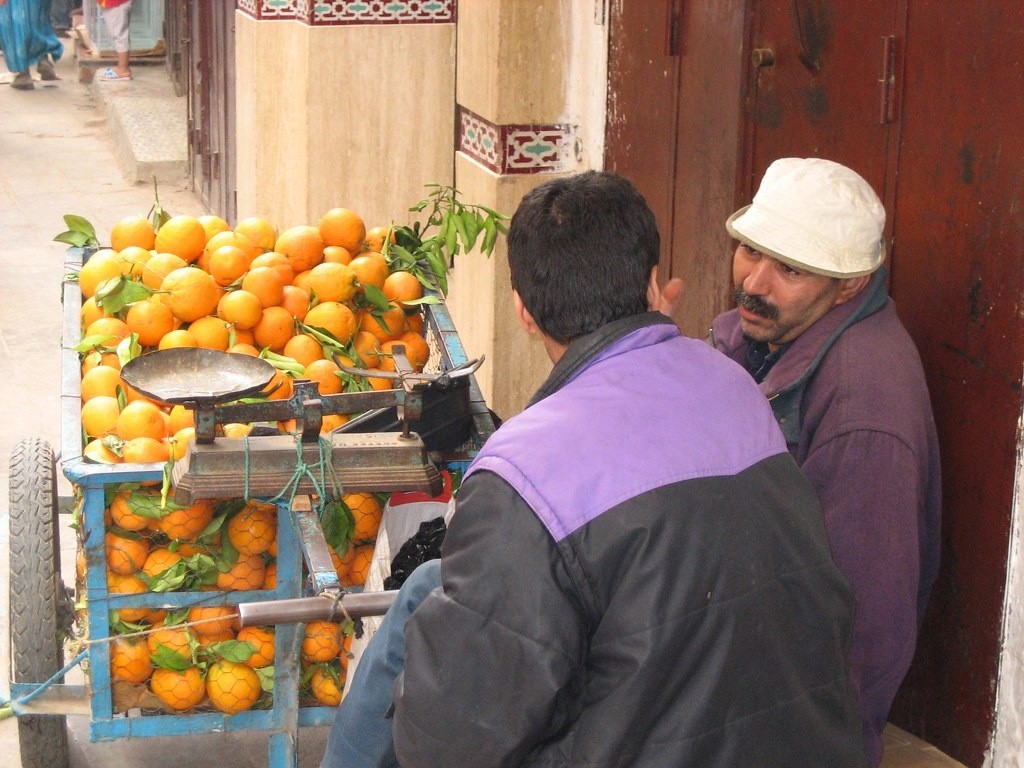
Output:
<box><xmin>10</xmin><ymin>74</ymin><xmax>34</xmax><ymax>90</ymax></box>
<box><xmin>37</xmin><ymin>60</ymin><xmax>56</xmax><ymax>80</ymax></box>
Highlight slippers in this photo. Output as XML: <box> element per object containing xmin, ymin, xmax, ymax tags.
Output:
<box><xmin>99</xmin><ymin>68</ymin><xmax>132</xmax><ymax>80</ymax></box>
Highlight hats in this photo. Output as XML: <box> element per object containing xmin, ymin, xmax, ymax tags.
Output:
<box><xmin>726</xmin><ymin>159</ymin><xmax>887</xmax><ymax>278</ymax></box>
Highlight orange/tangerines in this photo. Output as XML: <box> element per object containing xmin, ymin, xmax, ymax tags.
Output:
<box><xmin>75</xmin><ymin>209</ymin><xmax>432</xmax><ymax>716</ymax></box>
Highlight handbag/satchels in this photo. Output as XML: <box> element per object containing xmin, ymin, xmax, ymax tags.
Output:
<box><xmin>339</xmin><ymin>470</ymin><xmax>458</xmax><ymax>713</ymax></box>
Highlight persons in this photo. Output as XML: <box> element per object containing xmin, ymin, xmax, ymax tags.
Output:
<box><xmin>98</xmin><ymin>0</ymin><xmax>135</xmax><ymax>81</ymax></box>
<box><xmin>659</xmin><ymin>157</ymin><xmax>942</xmax><ymax>768</ymax></box>
<box><xmin>0</xmin><ymin>0</ymin><xmax>66</xmax><ymax>90</ymax></box>
<box><xmin>319</xmin><ymin>171</ymin><xmax>859</xmax><ymax>768</ymax></box>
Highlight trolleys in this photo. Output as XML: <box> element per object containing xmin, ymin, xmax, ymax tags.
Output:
<box><xmin>0</xmin><ymin>245</ymin><xmax>501</xmax><ymax>768</ymax></box>
